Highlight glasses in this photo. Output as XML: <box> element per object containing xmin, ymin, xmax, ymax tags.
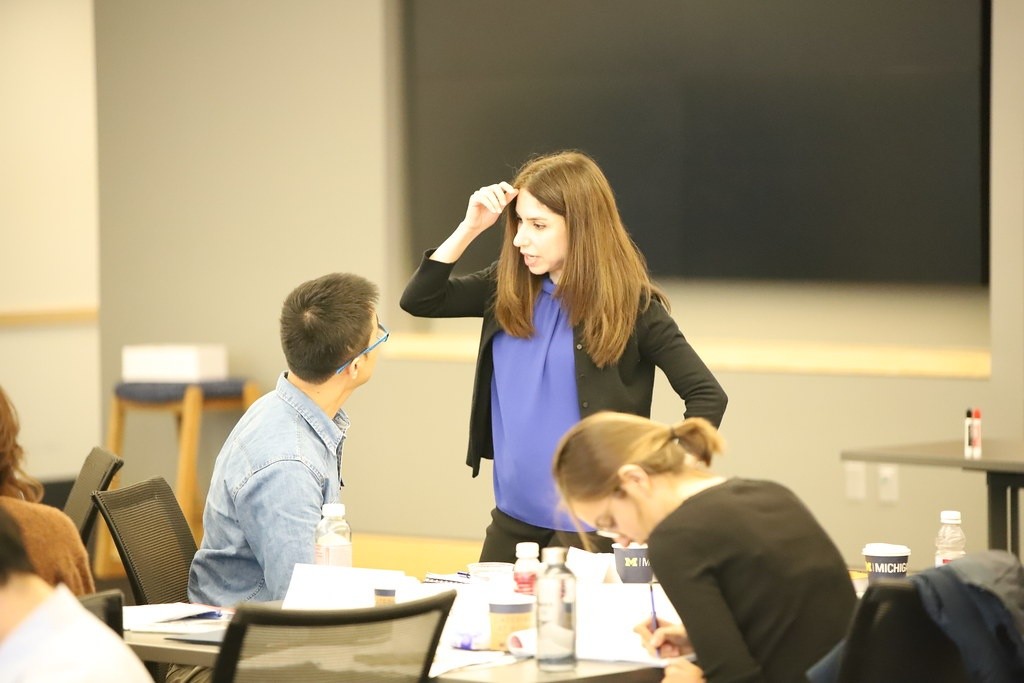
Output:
<box><xmin>596</xmin><ymin>483</ymin><xmax>620</xmax><ymax>538</ymax></box>
<box><xmin>337</xmin><ymin>322</ymin><xmax>389</xmax><ymax>375</ymax></box>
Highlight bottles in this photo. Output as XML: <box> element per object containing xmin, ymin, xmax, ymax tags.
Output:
<box><xmin>934</xmin><ymin>509</ymin><xmax>967</xmax><ymax>568</ymax></box>
<box><xmin>535</xmin><ymin>546</ymin><xmax>577</xmax><ymax>674</ymax></box>
<box><xmin>512</xmin><ymin>541</ymin><xmax>542</xmax><ymax>597</ymax></box>
<box><xmin>314</xmin><ymin>503</ymin><xmax>353</xmax><ymax>567</ymax></box>
<box><xmin>964</xmin><ymin>409</ymin><xmax>983</xmax><ymax>461</ymax></box>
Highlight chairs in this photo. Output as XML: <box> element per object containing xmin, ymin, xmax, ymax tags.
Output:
<box><xmin>91</xmin><ymin>473</ymin><xmax>198</xmax><ymax>683</ymax></box>
<box><xmin>836</xmin><ymin>549</ymin><xmax>1024</xmax><ymax>683</ymax></box>
<box><xmin>61</xmin><ymin>447</ymin><xmax>123</xmax><ymax>548</ymax></box>
<box><xmin>208</xmin><ymin>589</ymin><xmax>457</xmax><ymax>683</ymax></box>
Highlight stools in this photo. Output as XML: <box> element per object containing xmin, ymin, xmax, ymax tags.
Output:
<box><xmin>92</xmin><ymin>378</ymin><xmax>255</xmax><ymax>581</ymax></box>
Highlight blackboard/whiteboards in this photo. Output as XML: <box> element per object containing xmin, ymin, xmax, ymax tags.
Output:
<box><xmin>400</xmin><ymin>0</ymin><xmax>990</xmax><ymax>291</ymax></box>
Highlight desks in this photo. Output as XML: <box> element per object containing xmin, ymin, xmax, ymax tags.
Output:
<box><xmin>122</xmin><ymin>561</ymin><xmax>708</xmax><ymax>683</ymax></box>
<box><xmin>839</xmin><ymin>436</ymin><xmax>1024</xmax><ymax>560</ymax></box>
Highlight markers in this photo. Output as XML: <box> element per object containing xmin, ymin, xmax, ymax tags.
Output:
<box><xmin>973</xmin><ymin>411</ymin><xmax>982</xmax><ymax>459</ymax></box>
<box><xmin>965</xmin><ymin>411</ymin><xmax>973</xmax><ymax>458</ymax></box>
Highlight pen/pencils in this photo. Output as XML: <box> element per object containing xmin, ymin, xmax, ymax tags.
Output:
<box><xmin>649</xmin><ymin>586</ymin><xmax>661</xmax><ymax>659</ymax></box>
<box><xmin>187</xmin><ymin>612</ymin><xmax>222</xmax><ymax>618</ymax></box>
<box><xmin>457</xmin><ymin>571</ymin><xmax>471</xmax><ymax>578</ymax></box>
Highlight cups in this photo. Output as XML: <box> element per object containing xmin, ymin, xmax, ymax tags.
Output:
<box><xmin>861</xmin><ymin>542</ymin><xmax>911</xmax><ymax>585</ymax></box>
<box><xmin>611</xmin><ymin>541</ymin><xmax>653</xmax><ymax>582</ymax></box>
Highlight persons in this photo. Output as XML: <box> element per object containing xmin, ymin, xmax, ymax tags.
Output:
<box><xmin>551</xmin><ymin>410</ymin><xmax>857</xmax><ymax>683</ymax></box>
<box><xmin>0</xmin><ymin>504</ymin><xmax>156</xmax><ymax>683</ymax></box>
<box><xmin>399</xmin><ymin>151</ymin><xmax>729</xmax><ymax>562</ymax></box>
<box><xmin>186</xmin><ymin>274</ymin><xmax>389</xmax><ymax>609</ymax></box>
<box><xmin>0</xmin><ymin>382</ymin><xmax>96</xmax><ymax>597</ymax></box>
